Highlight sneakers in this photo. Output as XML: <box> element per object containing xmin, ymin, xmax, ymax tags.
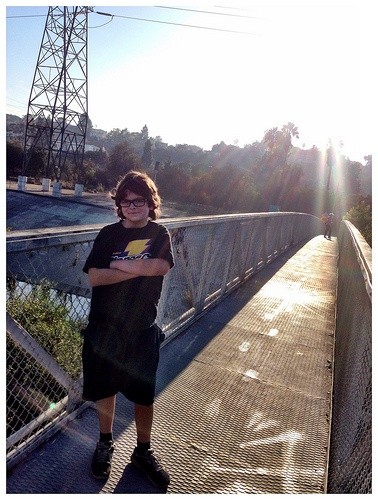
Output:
<box><xmin>92</xmin><ymin>440</ymin><xmax>116</xmax><ymax>480</ymax></box>
<box><xmin>131</xmin><ymin>447</ymin><xmax>171</xmax><ymax>485</ymax></box>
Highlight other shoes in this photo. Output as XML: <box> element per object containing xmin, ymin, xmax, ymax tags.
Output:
<box><xmin>324</xmin><ymin>236</ymin><xmax>331</xmax><ymax>240</ymax></box>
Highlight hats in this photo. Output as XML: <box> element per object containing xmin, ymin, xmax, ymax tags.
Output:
<box><xmin>329</xmin><ymin>213</ymin><xmax>335</xmax><ymax>216</ymax></box>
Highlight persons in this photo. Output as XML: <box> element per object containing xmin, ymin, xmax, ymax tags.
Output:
<box><xmin>324</xmin><ymin>213</ymin><xmax>335</xmax><ymax>240</ymax></box>
<box><xmin>72</xmin><ymin>171</ymin><xmax>173</xmax><ymax>487</ymax></box>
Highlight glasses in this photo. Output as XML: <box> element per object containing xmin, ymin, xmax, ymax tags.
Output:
<box><xmin>115</xmin><ymin>198</ymin><xmax>148</xmax><ymax>207</ymax></box>
